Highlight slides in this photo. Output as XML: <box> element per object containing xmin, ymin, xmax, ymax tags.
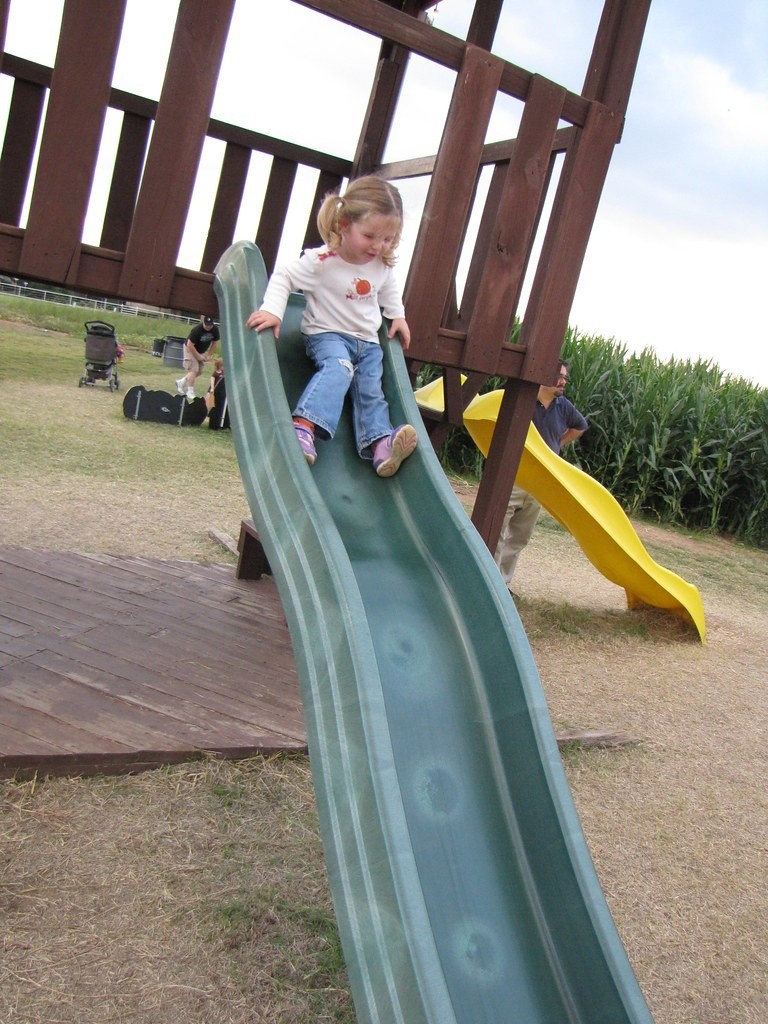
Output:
<box><xmin>415</xmin><ymin>372</ymin><xmax>482</xmax><ymax>410</ymax></box>
<box><xmin>211</xmin><ymin>235</ymin><xmax>659</xmax><ymax>1023</ymax></box>
<box><xmin>460</xmin><ymin>384</ymin><xmax>712</xmax><ymax>643</ymax></box>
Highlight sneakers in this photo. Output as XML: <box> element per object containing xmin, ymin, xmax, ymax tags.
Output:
<box><xmin>294</xmin><ymin>423</ymin><xmax>318</xmax><ymax>465</ymax></box>
<box><xmin>373</xmin><ymin>424</ymin><xmax>417</xmax><ymax>478</ymax></box>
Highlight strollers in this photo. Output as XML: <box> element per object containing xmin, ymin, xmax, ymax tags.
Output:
<box><xmin>78</xmin><ymin>321</ymin><xmax>120</xmax><ymax>393</ymax></box>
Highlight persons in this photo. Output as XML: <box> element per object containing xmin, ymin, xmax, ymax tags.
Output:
<box><xmin>205</xmin><ymin>361</ymin><xmax>225</xmax><ymax>414</ymax></box>
<box><xmin>176</xmin><ymin>316</ymin><xmax>221</xmax><ymax>401</ymax></box>
<box><xmin>245</xmin><ymin>175</ymin><xmax>418</xmax><ymax>478</ymax></box>
<box><xmin>484</xmin><ymin>356</ymin><xmax>586</xmax><ymax>603</ymax></box>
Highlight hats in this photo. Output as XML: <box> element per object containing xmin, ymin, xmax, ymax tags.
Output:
<box><xmin>204</xmin><ymin>317</ymin><xmax>213</xmax><ymax>325</ymax></box>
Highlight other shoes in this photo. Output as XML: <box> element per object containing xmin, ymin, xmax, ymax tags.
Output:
<box><xmin>175</xmin><ymin>380</ymin><xmax>184</xmax><ymax>394</ymax></box>
<box><xmin>186</xmin><ymin>392</ymin><xmax>196</xmax><ymax>399</ymax></box>
